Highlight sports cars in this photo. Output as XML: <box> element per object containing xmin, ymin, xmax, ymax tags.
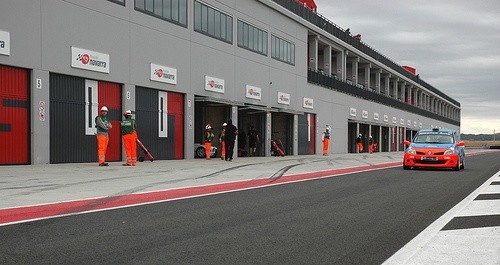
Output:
<box><xmin>402</xmin><ymin>128</ymin><xmax>466</xmax><ymax>171</ymax></box>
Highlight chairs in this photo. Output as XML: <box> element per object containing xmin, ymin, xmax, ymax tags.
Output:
<box><xmin>439</xmin><ymin>136</ymin><xmax>453</xmax><ymax>144</ymax></box>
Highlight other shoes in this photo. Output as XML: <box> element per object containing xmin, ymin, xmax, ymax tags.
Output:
<box><xmin>221</xmin><ymin>157</ymin><xmax>224</xmax><ymax>160</ymax></box>
<box><xmin>99</xmin><ymin>162</ymin><xmax>108</xmax><ymax>166</ymax></box>
<box><xmin>249</xmin><ymin>155</ymin><xmax>252</xmax><ymax>157</ymax></box>
<box><xmin>225</xmin><ymin>158</ymin><xmax>233</xmax><ymax>161</ymax></box>
<box><xmin>122</xmin><ymin>163</ymin><xmax>135</xmax><ymax>166</ymax></box>
<box><xmin>206</xmin><ymin>158</ymin><xmax>211</xmax><ymax>160</ymax></box>
<box><xmin>253</xmin><ymin>152</ymin><xmax>255</xmax><ymax>157</ymax></box>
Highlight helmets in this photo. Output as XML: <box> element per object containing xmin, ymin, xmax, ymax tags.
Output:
<box><xmin>326</xmin><ymin>128</ymin><xmax>329</xmax><ymax>130</ymax></box>
<box><xmin>206</xmin><ymin>124</ymin><xmax>213</xmax><ymax>129</ymax></box>
<box><xmin>123</xmin><ymin>110</ymin><xmax>132</xmax><ymax>116</ymax></box>
<box><xmin>101</xmin><ymin>106</ymin><xmax>108</xmax><ymax>111</ymax></box>
<box><xmin>369</xmin><ymin>135</ymin><xmax>372</xmax><ymax>138</ymax></box>
<box><xmin>360</xmin><ymin>134</ymin><xmax>362</xmax><ymax>136</ymax></box>
<box><xmin>223</xmin><ymin>123</ymin><xmax>227</xmax><ymax>126</ymax></box>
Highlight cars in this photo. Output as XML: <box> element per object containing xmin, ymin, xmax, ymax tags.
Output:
<box><xmin>194</xmin><ymin>143</ymin><xmax>218</xmax><ymax>159</ymax></box>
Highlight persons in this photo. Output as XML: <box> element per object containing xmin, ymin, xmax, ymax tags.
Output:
<box><xmin>356</xmin><ymin>134</ymin><xmax>363</xmax><ymax>153</ymax></box>
<box><xmin>120</xmin><ymin>110</ymin><xmax>138</xmax><ymax>166</ymax></box>
<box><xmin>368</xmin><ymin>135</ymin><xmax>374</xmax><ymax>153</ymax></box>
<box><xmin>225</xmin><ymin>120</ymin><xmax>238</xmax><ymax>161</ymax></box>
<box><xmin>345</xmin><ymin>28</ymin><xmax>350</xmax><ymax>33</ymax></box>
<box><xmin>219</xmin><ymin>123</ymin><xmax>227</xmax><ymax>160</ymax></box>
<box><xmin>247</xmin><ymin>125</ymin><xmax>259</xmax><ymax>157</ymax></box>
<box><xmin>417</xmin><ymin>74</ymin><xmax>420</xmax><ymax>77</ymax></box>
<box><xmin>204</xmin><ymin>124</ymin><xmax>214</xmax><ymax>160</ymax></box>
<box><xmin>95</xmin><ymin>106</ymin><xmax>112</xmax><ymax>166</ymax></box>
<box><xmin>353</xmin><ymin>34</ymin><xmax>361</xmax><ymax>41</ymax></box>
<box><xmin>322</xmin><ymin>127</ymin><xmax>330</xmax><ymax>156</ymax></box>
